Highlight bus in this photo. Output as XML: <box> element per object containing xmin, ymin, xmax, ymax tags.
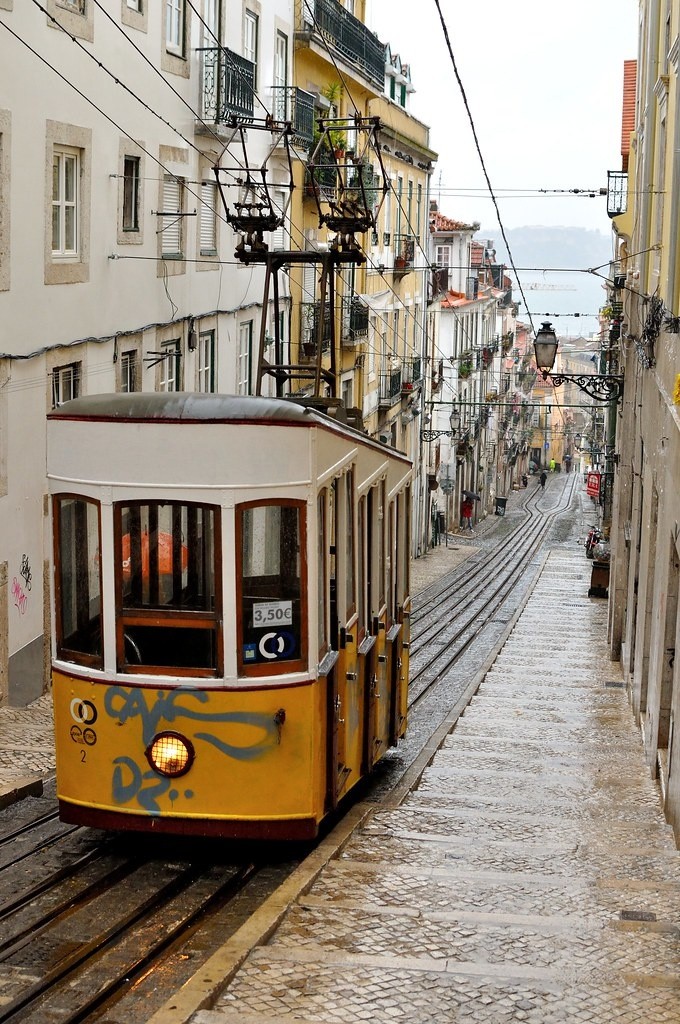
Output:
<box><xmin>43</xmin><ymin>391</ymin><xmax>413</xmax><ymax>844</ymax></box>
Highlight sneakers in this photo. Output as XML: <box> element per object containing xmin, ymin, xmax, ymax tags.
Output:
<box><xmin>459</xmin><ymin>526</ymin><xmax>464</xmax><ymax>530</ymax></box>
<box><xmin>470</xmin><ymin>529</ymin><xmax>475</xmax><ymax>533</ymax></box>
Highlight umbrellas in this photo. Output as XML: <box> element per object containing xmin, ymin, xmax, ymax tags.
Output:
<box><xmin>564</xmin><ymin>455</ymin><xmax>572</xmax><ymax>459</ymax></box>
<box><xmin>461</xmin><ymin>490</ymin><xmax>481</xmax><ymax>501</ymax></box>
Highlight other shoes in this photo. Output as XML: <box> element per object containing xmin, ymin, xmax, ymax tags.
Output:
<box><xmin>542</xmin><ymin>487</ymin><xmax>545</xmax><ymax>491</ymax></box>
<box><xmin>550</xmin><ymin>470</ymin><xmax>554</xmax><ymax>473</ymax></box>
<box><xmin>566</xmin><ymin>470</ymin><xmax>569</xmax><ymax>473</ymax></box>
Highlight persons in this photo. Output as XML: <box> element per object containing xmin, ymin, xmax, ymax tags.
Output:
<box><xmin>540</xmin><ymin>473</ymin><xmax>547</xmax><ymax>490</ymax></box>
<box><xmin>460</xmin><ymin>497</ymin><xmax>474</xmax><ymax>532</ymax></box>
<box><xmin>566</xmin><ymin>459</ymin><xmax>571</xmax><ymax>473</ymax></box>
<box><xmin>550</xmin><ymin>458</ymin><xmax>555</xmax><ymax>474</ymax></box>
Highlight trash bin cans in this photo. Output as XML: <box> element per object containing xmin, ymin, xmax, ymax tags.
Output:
<box><xmin>495</xmin><ymin>497</ymin><xmax>508</xmax><ymax>516</ymax></box>
<box><xmin>588</xmin><ymin>561</ymin><xmax>610</xmax><ymax>599</ymax></box>
<box><xmin>522</xmin><ymin>476</ymin><xmax>527</xmax><ymax>487</ymax></box>
<box><xmin>436</xmin><ymin>510</ymin><xmax>445</xmax><ymax>533</ymax></box>
